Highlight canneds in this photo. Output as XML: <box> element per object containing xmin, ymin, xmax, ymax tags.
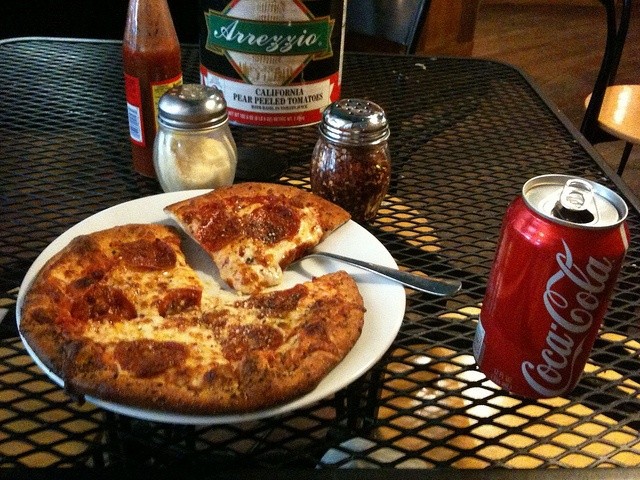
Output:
<box><xmin>469</xmin><ymin>174</ymin><xmax>629</xmax><ymax>399</ymax></box>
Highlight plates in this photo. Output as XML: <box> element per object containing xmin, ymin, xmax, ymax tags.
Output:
<box><xmin>16</xmin><ymin>188</ymin><xmax>408</xmax><ymax>426</ymax></box>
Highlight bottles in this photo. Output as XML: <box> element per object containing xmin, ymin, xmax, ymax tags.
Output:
<box><xmin>312</xmin><ymin>97</ymin><xmax>392</xmax><ymax>225</ymax></box>
<box><xmin>125</xmin><ymin>0</ymin><xmax>183</xmax><ymax>177</ymax></box>
<box><xmin>153</xmin><ymin>83</ymin><xmax>237</xmax><ymax>190</ymax></box>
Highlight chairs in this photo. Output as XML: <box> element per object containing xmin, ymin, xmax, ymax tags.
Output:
<box><xmin>278</xmin><ymin>0</ymin><xmax>432</xmax><ymax>192</ymax></box>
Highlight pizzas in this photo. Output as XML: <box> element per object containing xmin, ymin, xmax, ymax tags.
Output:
<box><xmin>19</xmin><ymin>183</ymin><xmax>367</xmax><ymax>417</ymax></box>
